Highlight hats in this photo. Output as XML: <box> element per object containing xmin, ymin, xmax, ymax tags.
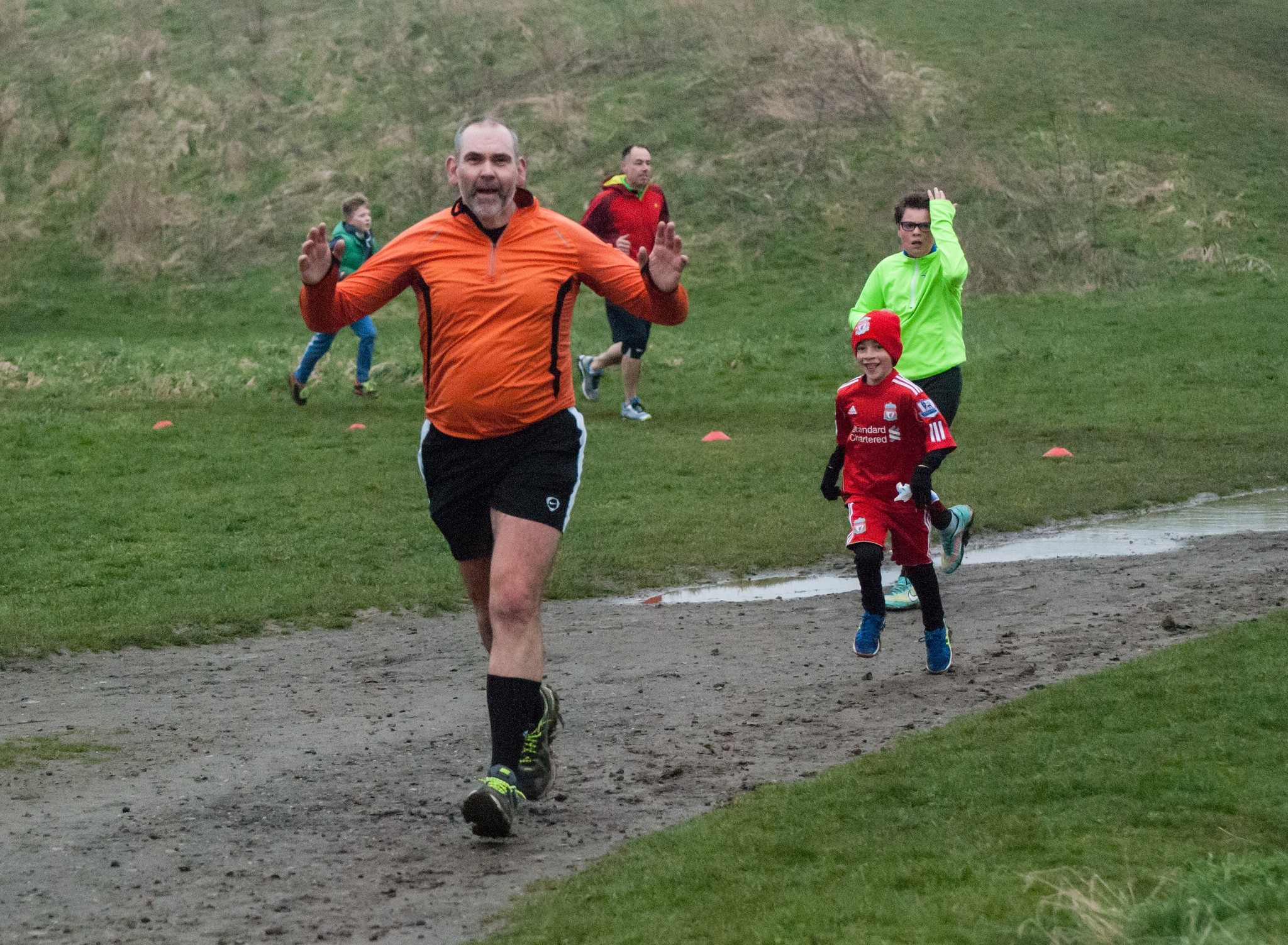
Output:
<box><xmin>851</xmin><ymin>309</ymin><xmax>904</xmax><ymax>366</ymax></box>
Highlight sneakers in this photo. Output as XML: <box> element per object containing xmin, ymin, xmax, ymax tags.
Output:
<box><xmin>941</xmin><ymin>504</ymin><xmax>974</xmax><ymax>576</ymax></box>
<box><xmin>923</xmin><ymin>620</ymin><xmax>952</xmax><ymax>674</ymax></box>
<box><xmin>884</xmin><ymin>577</ymin><xmax>920</xmax><ymax>610</ymax></box>
<box><xmin>854</xmin><ymin>609</ymin><xmax>884</xmax><ymax>658</ymax></box>
<box><xmin>290</xmin><ymin>371</ymin><xmax>308</xmax><ymax>406</ymax></box>
<box><xmin>354</xmin><ymin>380</ymin><xmax>380</xmax><ymax>399</ymax></box>
<box><xmin>578</xmin><ymin>355</ymin><xmax>605</xmax><ymax>402</ymax></box>
<box><xmin>464</xmin><ymin>763</ymin><xmax>513</xmax><ymax>838</ymax></box>
<box><xmin>620</xmin><ymin>396</ymin><xmax>653</xmax><ymax>422</ymax></box>
<box><xmin>515</xmin><ymin>687</ymin><xmax>560</xmax><ymax>803</ymax></box>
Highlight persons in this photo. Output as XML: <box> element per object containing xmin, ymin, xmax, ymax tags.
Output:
<box><xmin>819</xmin><ymin>309</ymin><xmax>958</xmax><ymax>673</ymax></box>
<box><xmin>287</xmin><ymin>192</ymin><xmax>378</xmax><ymax>406</ymax></box>
<box><xmin>848</xmin><ymin>185</ymin><xmax>975</xmax><ymax>613</ymax></box>
<box><xmin>576</xmin><ymin>143</ymin><xmax>669</xmax><ymax>422</ymax></box>
<box><xmin>297</xmin><ymin>117</ymin><xmax>689</xmax><ymax>838</ymax></box>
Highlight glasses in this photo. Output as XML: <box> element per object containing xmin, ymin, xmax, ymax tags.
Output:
<box><xmin>898</xmin><ymin>220</ymin><xmax>933</xmax><ymax>233</ymax></box>
